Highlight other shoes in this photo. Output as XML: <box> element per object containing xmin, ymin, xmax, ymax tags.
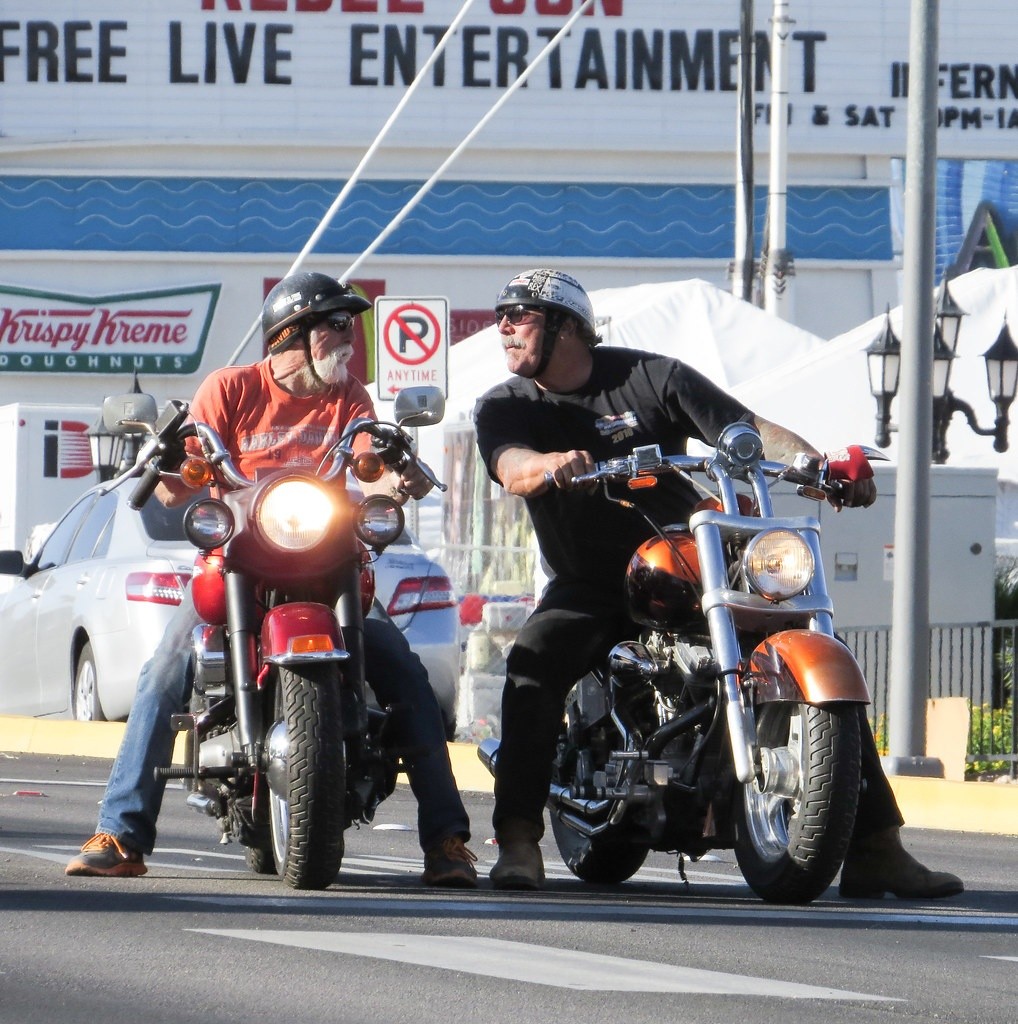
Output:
<box><xmin>837</xmin><ymin>824</ymin><xmax>964</xmax><ymax>900</ymax></box>
<box><xmin>490</xmin><ymin>834</ymin><xmax>545</xmax><ymax>888</ymax></box>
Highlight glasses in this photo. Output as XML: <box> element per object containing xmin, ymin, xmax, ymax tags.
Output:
<box><xmin>494</xmin><ymin>306</ymin><xmax>548</xmax><ymax>330</ymax></box>
<box><xmin>323</xmin><ymin>312</ymin><xmax>355</xmax><ymax>332</ymax></box>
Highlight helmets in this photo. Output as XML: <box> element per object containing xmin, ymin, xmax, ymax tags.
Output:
<box><xmin>498</xmin><ymin>268</ymin><xmax>596</xmax><ymax>333</ymax></box>
<box><xmin>261</xmin><ymin>271</ymin><xmax>371</xmax><ymax>343</ymax></box>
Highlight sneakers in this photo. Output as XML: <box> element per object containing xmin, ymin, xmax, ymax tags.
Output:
<box><xmin>423</xmin><ymin>834</ymin><xmax>479</xmax><ymax>888</ymax></box>
<box><xmin>64</xmin><ymin>833</ymin><xmax>148</xmax><ymax>878</ymax></box>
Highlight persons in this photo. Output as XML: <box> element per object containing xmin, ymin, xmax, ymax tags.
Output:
<box><xmin>476</xmin><ymin>268</ymin><xmax>966</xmax><ymax>903</ymax></box>
<box><xmin>61</xmin><ymin>268</ymin><xmax>481</xmax><ymax>883</ymax></box>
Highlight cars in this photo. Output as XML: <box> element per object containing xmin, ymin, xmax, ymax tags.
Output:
<box><xmin>0</xmin><ymin>481</ymin><xmax>464</xmax><ymax>744</ymax></box>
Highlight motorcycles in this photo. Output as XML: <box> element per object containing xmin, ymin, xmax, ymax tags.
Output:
<box><xmin>478</xmin><ymin>421</ymin><xmax>886</xmax><ymax>907</ymax></box>
<box><xmin>83</xmin><ymin>381</ymin><xmax>449</xmax><ymax>891</ymax></box>
